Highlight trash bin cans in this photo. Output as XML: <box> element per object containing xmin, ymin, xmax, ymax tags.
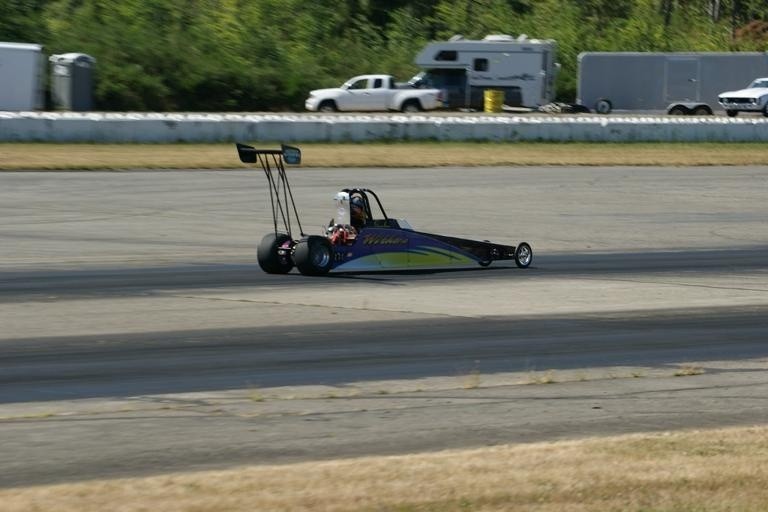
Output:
<box><xmin>484</xmin><ymin>89</ymin><xmax>505</xmax><ymax>113</ymax></box>
<box><xmin>50</xmin><ymin>53</ymin><xmax>97</xmax><ymax>111</ymax></box>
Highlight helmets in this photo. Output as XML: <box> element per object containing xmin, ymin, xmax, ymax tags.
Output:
<box><xmin>352</xmin><ymin>196</ymin><xmax>364</xmax><ymax>210</ymax></box>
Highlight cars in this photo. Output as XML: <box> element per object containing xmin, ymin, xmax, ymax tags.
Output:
<box><xmin>719</xmin><ymin>77</ymin><xmax>768</xmax><ymax>118</ymax></box>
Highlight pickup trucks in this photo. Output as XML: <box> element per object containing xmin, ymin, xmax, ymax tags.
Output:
<box><xmin>394</xmin><ymin>67</ymin><xmax>527</xmax><ymax>113</ymax></box>
<box><xmin>306</xmin><ymin>72</ymin><xmax>445</xmax><ymax>116</ymax></box>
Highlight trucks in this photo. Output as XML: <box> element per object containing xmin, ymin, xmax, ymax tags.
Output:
<box><xmin>406</xmin><ymin>33</ymin><xmax>558</xmax><ymax>111</ymax></box>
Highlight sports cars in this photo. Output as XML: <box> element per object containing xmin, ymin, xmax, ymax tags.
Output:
<box><xmin>231</xmin><ymin>137</ymin><xmax>535</xmax><ymax>278</ymax></box>
